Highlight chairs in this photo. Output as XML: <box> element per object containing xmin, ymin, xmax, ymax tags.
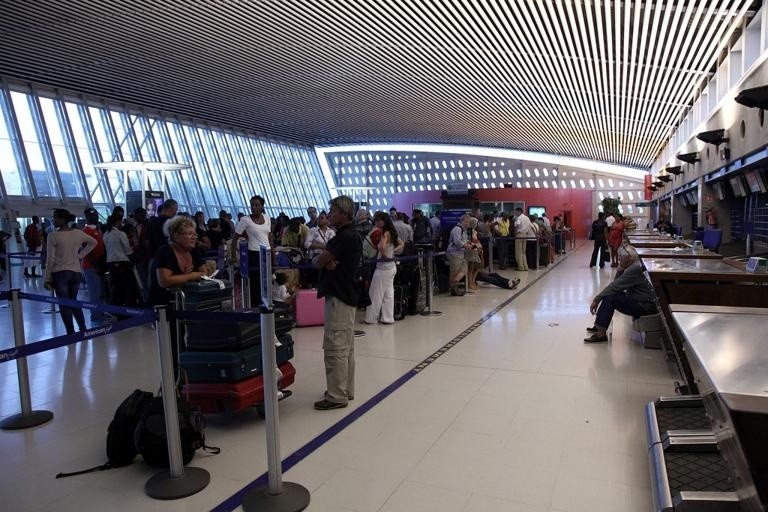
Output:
<box><xmin>646</xmin><ymin>221</ymin><xmax>721</xmax><ymax>252</ymax></box>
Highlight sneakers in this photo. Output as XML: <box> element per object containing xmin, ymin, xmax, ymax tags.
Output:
<box><xmin>584</xmin><ymin>326</ymin><xmax>608</xmax><ymax>343</ymax></box>
<box><xmin>314</xmin><ymin>391</ymin><xmax>353</xmax><ymax>409</ymax></box>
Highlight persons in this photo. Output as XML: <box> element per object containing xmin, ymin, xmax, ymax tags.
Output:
<box><xmin>446</xmin><ymin>207</ymin><xmax>566</xmax><ymax>297</ymax></box>
<box><xmin>1</xmin><ymin>216</ymin><xmax>52</xmax><ymax>279</ymax></box>
<box><xmin>582</xmin><ymin>243</ymin><xmax>658</xmax><ymax>343</ymax></box>
<box><xmin>356</xmin><ymin>207</ymin><xmax>441</xmax><ymax>324</ymax></box>
<box><xmin>589</xmin><ymin>212</ymin><xmax>608</xmax><ymax>269</ymax></box>
<box><xmin>605</xmin><ymin>213</ymin><xmax>615</xmax><ymax>233</ymax></box>
<box><xmin>43</xmin><ymin>206</ymin><xmax>157</xmax><ymax>333</ymax></box>
<box><xmin>142</xmin><ymin>193</ymin><xmax>373</xmax><ymax>408</ymax></box>
<box><xmin>653</xmin><ymin>214</ymin><xmax>675</xmax><ymax>234</ymax></box>
<box><xmin>608</xmin><ymin>214</ymin><xmax>625</xmax><ymax>268</ymax></box>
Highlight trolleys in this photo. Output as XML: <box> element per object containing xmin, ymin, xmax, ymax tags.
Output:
<box><xmin>173</xmin><ymin>277</ymin><xmax>293</xmax><ymax>416</ymax></box>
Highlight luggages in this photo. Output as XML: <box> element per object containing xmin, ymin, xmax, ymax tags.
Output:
<box><xmin>178</xmin><ymin>277</ymin><xmax>295</xmax><ymax>414</ymax></box>
<box><xmin>294</xmin><ymin>288</ymin><xmax>327</xmax><ymax>327</ymax></box>
<box><xmin>404</xmin><ymin>248</ymin><xmax>428</xmax><ymax>315</ymax></box>
<box><xmin>394</xmin><ymin>283</ymin><xmax>410</xmax><ymax>320</ymax></box>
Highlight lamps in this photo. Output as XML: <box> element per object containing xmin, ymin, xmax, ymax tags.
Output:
<box><xmin>645</xmin><ymin>85</ymin><xmax>768</xmax><ymax>192</ymax></box>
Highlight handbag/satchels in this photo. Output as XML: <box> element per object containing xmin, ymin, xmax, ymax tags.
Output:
<box><xmin>347</xmin><ymin>265</ymin><xmax>372</xmax><ymax>308</ymax></box>
<box><xmin>107</xmin><ymin>389</ymin><xmax>205</xmax><ymax>467</ymax></box>
<box><xmin>605</xmin><ymin>251</ymin><xmax>610</xmax><ymax>262</ymax></box>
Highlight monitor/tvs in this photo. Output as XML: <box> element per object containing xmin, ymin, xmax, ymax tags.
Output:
<box><xmin>528</xmin><ymin>206</ymin><xmax>546</xmax><ymax>219</ymax></box>
